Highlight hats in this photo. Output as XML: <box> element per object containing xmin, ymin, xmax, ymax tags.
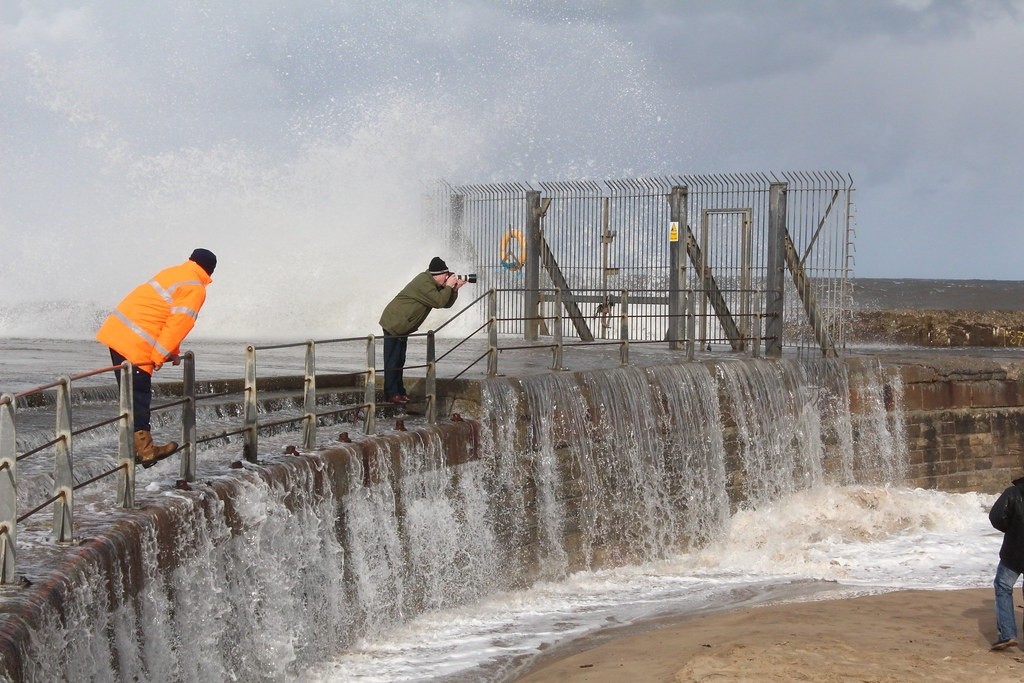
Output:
<box><xmin>429</xmin><ymin>257</ymin><xmax>449</xmax><ymax>275</ymax></box>
<box><xmin>189</xmin><ymin>249</ymin><xmax>217</xmax><ymax>276</ymax></box>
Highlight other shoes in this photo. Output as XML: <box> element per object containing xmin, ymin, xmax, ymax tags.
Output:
<box><xmin>991</xmin><ymin>639</ymin><xmax>1018</xmax><ymax>650</ymax></box>
<box><xmin>400</xmin><ymin>394</ymin><xmax>410</xmax><ymax>401</ymax></box>
<box><xmin>386</xmin><ymin>394</ymin><xmax>407</xmax><ymax>404</ymax></box>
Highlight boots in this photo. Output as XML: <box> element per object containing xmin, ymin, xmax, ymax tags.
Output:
<box><xmin>133</xmin><ymin>429</ymin><xmax>179</xmax><ymax>469</ymax></box>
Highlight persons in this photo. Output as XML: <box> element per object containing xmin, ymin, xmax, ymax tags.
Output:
<box><xmin>379</xmin><ymin>257</ymin><xmax>467</xmax><ymax>404</ymax></box>
<box><xmin>95</xmin><ymin>249</ymin><xmax>217</xmax><ymax>469</ymax></box>
<box><xmin>989</xmin><ymin>478</ymin><xmax>1024</xmax><ymax>650</ymax></box>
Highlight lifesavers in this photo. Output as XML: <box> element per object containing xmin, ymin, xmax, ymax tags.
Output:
<box><xmin>497</xmin><ymin>230</ymin><xmax>529</xmax><ymax>271</ymax></box>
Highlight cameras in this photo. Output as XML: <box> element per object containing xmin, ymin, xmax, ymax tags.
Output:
<box><xmin>447</xmin><ymin>272</ymin><xmax>477</xmax><ymax>284</ymax></box>
